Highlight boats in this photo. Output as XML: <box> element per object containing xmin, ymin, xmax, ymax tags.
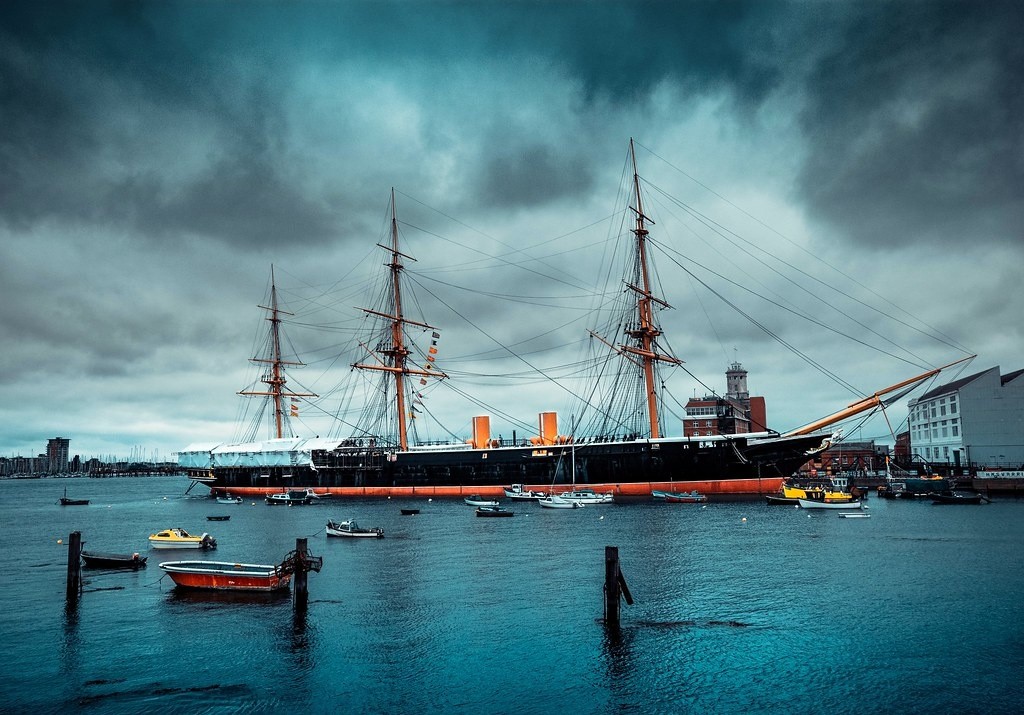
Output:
<box><xmin>876</xmin><ymin>480</ymin><xmax>935</xmax><ymax>499</ymax></box>
<box><xmin>652</xmin><ymin>489</ymin><xmax>689</xmax><ymax>499</ymax></box>
<box><xmin>266</xmin><ymin>488</ymin><xmax>333</xmax><ymax>506</ymax></box>
<box><xmin>503</xmin><ymin>483</ymin><xmax>559</xmax><ymax>501</ymax></box>
<box><xmin>560</xmin><ymin>490</ymin><xmax>614</xmax><ymax>504</ymax></box>
<box><xmin>80</xmin><ymin>549</ymin><xmax>149</xmax><ymax>569</ymax></box>
<box><xmin>932</xmin><ymin>492</ymin><xmax>992</xmax><ymax>504</ymax></box>
<box><xmin>149</xmin><ymin>528</ymin><xmax>217</xmax><ymax>550</ymax></box>
<box><xmin>781</xmin><ymin>472</ymin><xmax>834</xmax><ymax>498</ymax></box>
<box><xmin>475</xmin><ymin>506</ymin><xmax>514</xmax><ymax>517</ymax></box>
<box><xmin>400</xmin><ymin>508</ymin><xmax>420</xmax><ymax>515</ymax></box>
<box><xmin>60</xmin><ymin>496</ymin><xmax>89</xmax><ymax>505</ymax></box>
<box><xmin>325</xmin><ymin>520</ymin><xmax>384</xmax><ymax>539</ymax></box>
<box><xmin>664</xmin><ymin>490</ymin><xmax>708</xmax><ymax>502</ymax></box>
<box><xmin>797</xmin><ymin>489</ymin><xmax>861</xmax><ymax>509</ymax></box>
<box><xmin>158</xmin><ymin>557</ymin><xmax>292</xmax><ymax>592</ymax></box>
<box><xmin>766</xmin><ymin>493</ymin><xmax>800</xmax><ymax>505</ymax></box>
<box><xmin>216</xmin><ymin>494</ymin><xmax>243</xmax><ymax>504</ymax></box>
<box><xmin>177</xmin><ymin>135</ymin><xmax>980</xmax><ymax>507</ymax></box>
<box><xmin>463</xmin><ymin>494</ymin><xmax>500</xmax><ymax>506</ymax></box>
<box><xmin>207</xmin><ymin>515</ymin><xmax>230</xmax><ymax>520</ymax></box>
<box><xmin>536</xmin><ymin>496</ymin><xmax>585</xmax><ymax>510</ymax></box>
<box><xmin>839</xmin><ymin>511</ymin><xmax>870</xmax><ymax>517</ymax></box>
